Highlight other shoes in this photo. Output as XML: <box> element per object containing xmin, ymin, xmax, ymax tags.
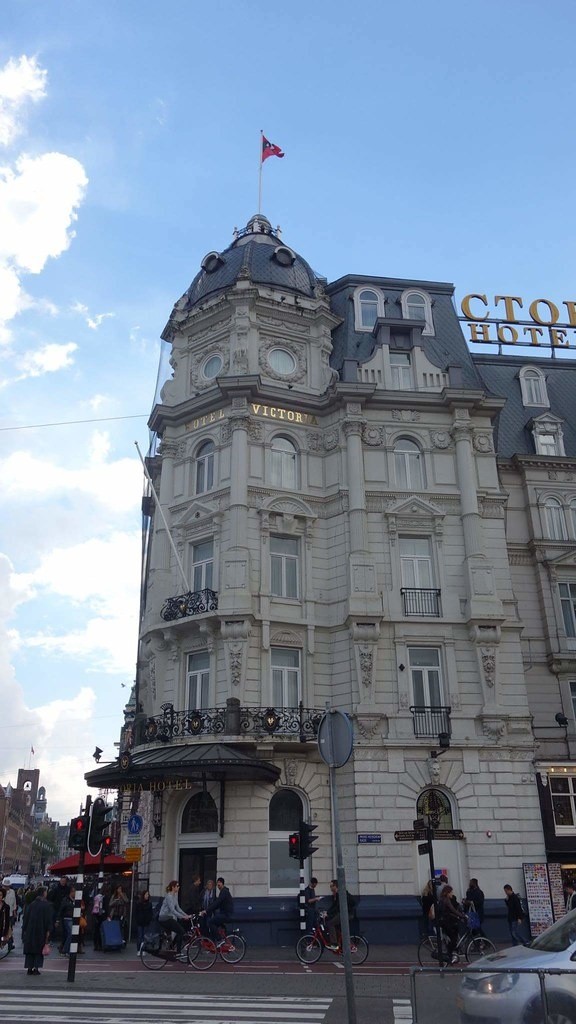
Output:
<box><xmin>8</xmin><ymin>945</ymin><xmax>15</xmax><ymax>949</ymax></box>
<box><xmin>137</xmin><ymin>950</ymin><xmax>141</xmax><ymax>956</ymax></box>
<box><xmin>33</xmin><ymin>969</ymin><xmax>41</xmax><ymax>975</ymax></box>
<box><xmin>324</xmin><ymin>943</ymin><xmax>340</xmax><ymax>950</ymax></box>
<box><xmin>175</xmin><ymin>952</ymin><xmax>188</xmax><ymax>960</ymax></box>
<box><xmin>142</xmin><ymin>951</ymin><xmax>146</xmax><ymax>957</ymax></box>
<box><xmin>61</xmin><ymin>953</ymin><xmax>69</xmax><ymax>957</ymax></box>
<box><xmin>27</xmin><ymin>969</ymin><xmax>34</xmax><ymax>975</ymax></box>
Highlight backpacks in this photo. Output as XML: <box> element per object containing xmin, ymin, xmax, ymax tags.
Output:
<box><xmin>298</xmin><ymin>891</ymin><xmax>307</xmax><ymax>911</ymax></box>
<box><xmin>516</xmin><ymin>894</ymin><xmax>527</xmax><ymax>914</ymax></box>
<box><xmin>429</xmin><ymin>901</ymin><xmax>436</xmax><ymax>920</ymax></box>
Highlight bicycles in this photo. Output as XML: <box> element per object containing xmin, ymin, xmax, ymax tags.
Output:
<box><xmin>418</xmin><ymin>919</ymin><xmax>496</xmax><ymax>967</ymax></box>
<box><xmin>140</xmin><ymin>914</ymin><xmax>247</xmax><ymax>970</ymax></box>
<box><xmin>295</xmin><ymin>911</ymin><xmax>369</xmax><ymax>965</ymax></box>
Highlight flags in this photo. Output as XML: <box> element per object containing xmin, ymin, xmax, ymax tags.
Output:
<box><xmin>262</xmin><ymin>134</ymin><xmax>284</xmax><ymax>163</ymax></box>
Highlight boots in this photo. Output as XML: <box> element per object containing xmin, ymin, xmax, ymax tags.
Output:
<box><xmin>78</xmin><ymin>943</ymin><xmax>85</xmax><ymax>954</ymax></box>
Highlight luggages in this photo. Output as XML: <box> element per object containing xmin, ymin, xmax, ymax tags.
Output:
<box><xmin>100</xmin><ymin>916</ymin><xmax>122</xmax><ymax>954</ymax></box>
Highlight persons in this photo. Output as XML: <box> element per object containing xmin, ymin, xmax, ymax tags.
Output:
<box><xmin>158</xmin><ymin>881</ymin><xmax>189</xmax><ymax>959</ymax></box>
<box><xmin>188</xmin><ymin>875</ymin><xmax>216</xmax><ymax>938</ymax></box>
<box><xmin>504</xmin><ymin>885</ymin><xmax>532</xmax><ymax>946</ymax></box>
<box><xmin>421</xmin><ymin>874</ymin><xmax>496</xmax><ymax>952</ymax></box>
<box><xmin>323</xmin><ymin>880</ymin><xmax>358</xmax><ymax>953</ymax></box>
<box><xmin>202</xmin><ymin>877</ymin><xmax>234</xmax><ymax>953</ymax></box>
<box><xmin>305</xmin><ymin>878</ymin><xmax>321</xmax><ymax>949</ymax></box>
<box><xmin>93</xmin><ymin>883</ymin><xmax>129</xmax><ymax>951</ymax></box>
<box><xmin>135</xmin><ymin>890</ymin><xmax>153</xmax><ymax>957</ymax></box>
<box><xmin>565</xmin><ymin>882</ymin><xmax>576</xmax><ymax>943</ymax></box>
<box><xmin>0</xmin><ymin>876</ymin><xmax>88</xmax><ymax>976</ymax></box>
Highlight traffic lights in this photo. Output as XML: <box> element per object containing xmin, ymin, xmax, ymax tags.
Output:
<box><xmin>87</xmin><ymin>798</ymin><xmax>114</xmax><ymax>858</ymax></box>
<box><xmin>288</xmin><ymin>835</ymin><xmax>300</xmax><ymax>859</ymax></box>
<box><xmin>301</xmin><ymin>820</ymin><xmax>319</xmax><ymax>859</ymax></box>
<box><xmin>69</xmin><ymin>816</ymin><xmax>89</xmax><ymax>850</ymax></box>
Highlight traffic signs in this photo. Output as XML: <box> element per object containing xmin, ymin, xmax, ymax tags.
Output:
<box><xmin>394</xmin><ymin>818</ymin><xmax>464</xmax><ymax>856</ymax></box>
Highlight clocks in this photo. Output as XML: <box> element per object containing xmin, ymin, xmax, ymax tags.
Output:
<box><xmin>23</xmin><ymin>780</ymin><xmax>32</xmax><ymax>791</ymax></box>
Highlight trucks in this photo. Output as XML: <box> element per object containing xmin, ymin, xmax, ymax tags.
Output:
<box><xmin>2</xmin><ymin>875</ymin><xmax>28</xmax><ymax>890</ymax></box>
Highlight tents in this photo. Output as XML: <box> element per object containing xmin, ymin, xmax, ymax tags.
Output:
<box><xmin>47</xmin><ymin>850</ymin><xmax>133</xmax><ymax>875</ymax></box>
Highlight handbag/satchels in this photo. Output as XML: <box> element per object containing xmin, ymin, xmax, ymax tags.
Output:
<box><xmin>43</xmin><ymin>936</ymin><xmax>52</xmax><ymax>956</ymax></box>
<box><xmin>466</xmin><ymin>902</ymin><xmax>480</xmax><ymax>930</ymax></box>
<box><xmin>141</xmin><ymin>923</ymin><xmax>163</xmax><ymax>953</ymax></box>
<box><xmin>79</xmin><ymin>916</ymin><xmax>87</xmax><ymax>928</ymax></box>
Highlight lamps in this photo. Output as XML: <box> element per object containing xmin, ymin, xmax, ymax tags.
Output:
<box><xmin>299</xmin><ymin>717</ymin><xmax>320</xmax><ymax>743</ymax></box>
<box><xmin>431</xmin><ymin>733</ymin><xmax>451</xmax><ymax>758</ymax></box>
<box><xmin>93</xmin><ymin>746</ymin><xmax>119</xmax><ymax>763</ymax></box>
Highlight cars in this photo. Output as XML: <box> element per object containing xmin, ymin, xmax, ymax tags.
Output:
<box><xmin>455</xmin><ymin>908</ymin><xmax>576</xmax><ymax>1024</ymax></box>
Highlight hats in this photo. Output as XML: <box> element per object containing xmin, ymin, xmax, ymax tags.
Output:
<box><xmin>2</xmin><ymin>878</ymin><xmax>11</xmax><ymax>886</ymax></box>
<box><xmin>218</xmin><ymin>877</ymin><xmax>224</xmax><ymax>884</ymax></box>
